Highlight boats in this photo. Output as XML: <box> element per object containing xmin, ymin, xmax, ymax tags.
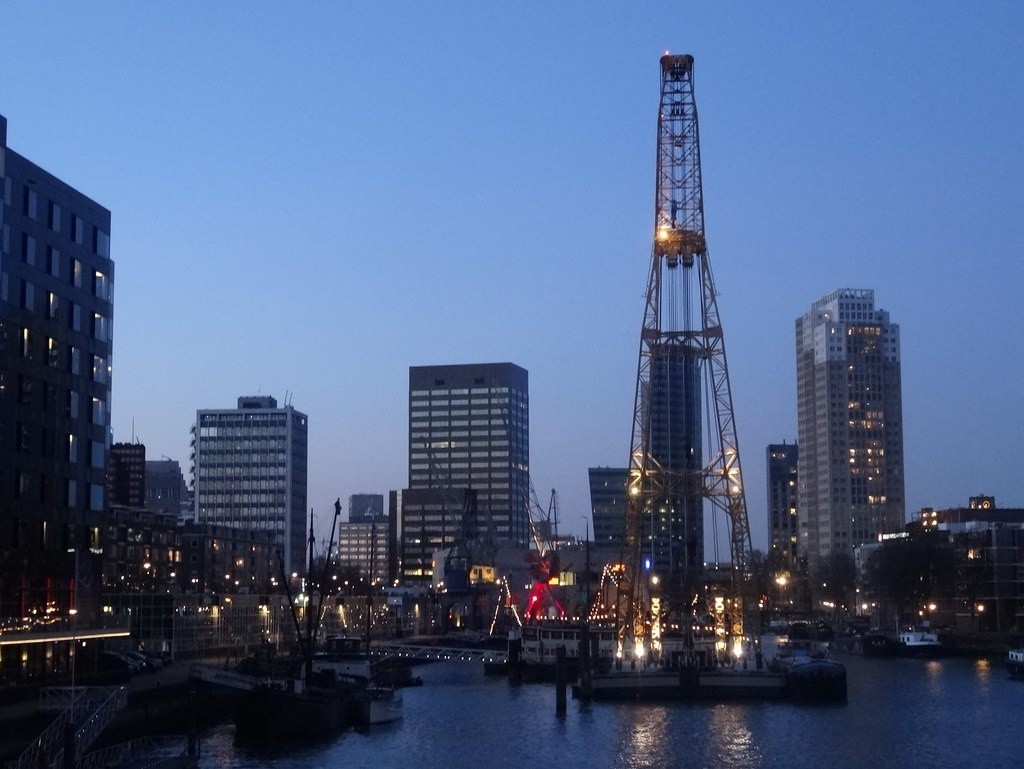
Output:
<box><xmin>302</xmin><ymin>633</ymin><xmax>373</xmax><ymax>686</ymax></box>
<box><xmin>342</xmin><ymin>684</ymin><xmax>406</xmax><ymax>730</ymax></box>
<box><xmin>1006</xmin><ymin>647</ymin><xmax>1024</xmax><ymax>676</ymax></box>
<box><xmin>768</xmin><ymin>654</ymin><xmax>848</xmax><ymax>702</ymax></box>
<box><xmin>895</xmin><ymin>631</ymin><xmax>947</xmax><ymax>660</ymax></box>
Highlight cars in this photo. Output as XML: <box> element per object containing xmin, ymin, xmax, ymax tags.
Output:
<box><xmin>81</xmin><ymin>652</ymin><xmax>172</xmax><ymax>677</ymax></box>
